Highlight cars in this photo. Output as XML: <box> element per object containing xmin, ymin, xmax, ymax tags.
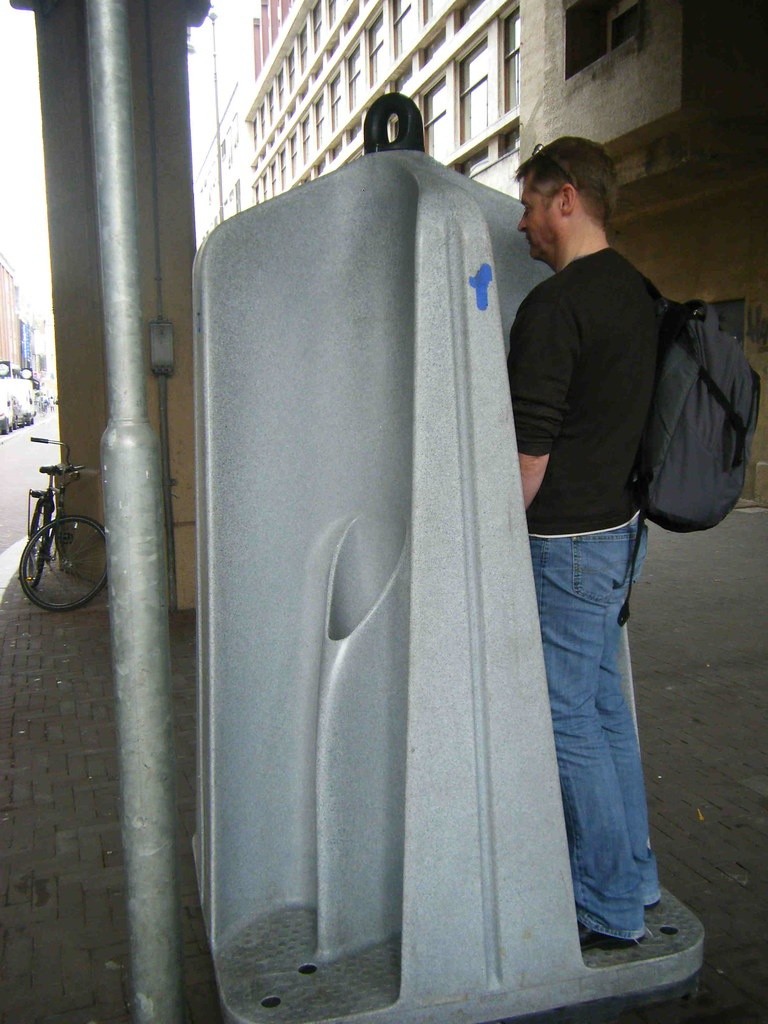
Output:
<box><xmin>0</xmin><ymin>378</ymin><xmax>37</xmax><ymax>434</ymax></box>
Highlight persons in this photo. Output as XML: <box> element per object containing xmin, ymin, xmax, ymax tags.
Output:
<box><xmin>508</xmin><ymin>137</ymin><xmax>660</xmax><ymax>953</ymax></box>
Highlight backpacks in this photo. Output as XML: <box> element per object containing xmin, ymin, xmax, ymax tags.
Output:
<box><xmin>618</xmin><ymin>271</ymin><xmax>761</xmax><ymax>626</ymax></box>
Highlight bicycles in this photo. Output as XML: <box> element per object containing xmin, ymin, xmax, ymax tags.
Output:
<box><xmin>18</xmin><ymin>438</ymin><xmax>110</xmax><ymax>613</ymax></box>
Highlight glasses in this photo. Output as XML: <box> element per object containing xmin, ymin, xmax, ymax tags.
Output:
<box><xmin>532</xmin><ymin>144</ymin><xmax>577</xmax><ymax>188</ymax></box>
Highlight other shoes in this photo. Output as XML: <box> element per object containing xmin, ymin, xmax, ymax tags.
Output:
<box><xmin>644</xmin><ymin>899</ymin><xmax>660</xmax><ymax>910</ymax></box>
<box><xmin>576</xmin><ymin>918</ymin><xmax>637</xmax><ymax>952</ymax></box>
